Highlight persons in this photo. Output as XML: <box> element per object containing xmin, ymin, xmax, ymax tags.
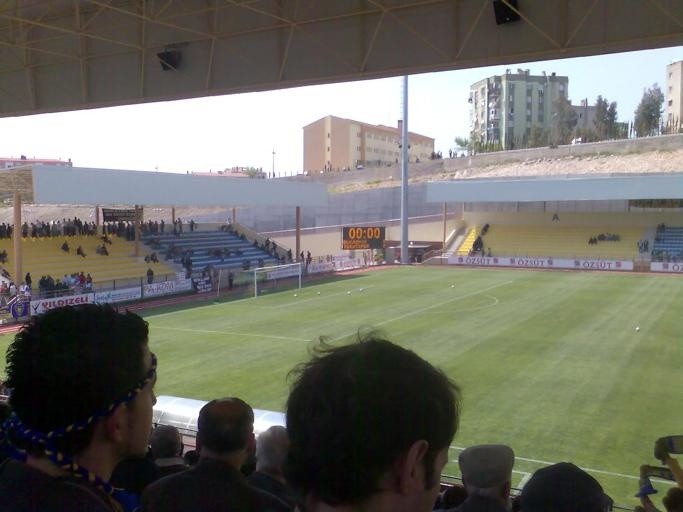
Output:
<box><xmin>2</xmin><ymin>403</ymin><xmax>26</xmax><ymax>450</ymax></box>
<box><xmin>111</xmin><ymin>396</ymin><xmax>299</xmax><ymax>511</ymax></box>
<box><xmin>306</xmin><ymin>250</ymin><xmax>310</xmax><ymax>258</ymax></box>
<box><xmin>300</xmin><ymin>249</ymin><xmax>304</xmax><ymax>261</ymax></box>
<box><xmin>587</xmin><ymin>232</ymin><xmax>620</xmax><ymax>246</ymax></box>
<box><xmin>283</xmin><ymin>338</ymin><xmax>458</xmax><ymax>512</ymax></box>
<box><xmin>0</xmin><ymin>303</ymin><xmax>156</xmax><ymax>512</ymax></box>
<box><xmin>435</xmin><ymin>443</ymin><xmax>515</xmax><ymax>511</ymax></box>
<box><xmin>634</xmin><ymin>438</ymin><xmax>682</xmax><ymax>511</ymax></box>
<box><xmin>0</xmin><ymin>213</ymin><xmax>294</xmax><ymax>307</ymax></box>
<box><xmin>638</xmin><ymin>222</ymin><xmax>682</xmax><ymax>262</ymax></box>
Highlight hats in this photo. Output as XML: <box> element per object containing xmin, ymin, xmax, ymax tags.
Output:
<box><xmin>458</xmin><ymin>443</ymin><xmax>514</xmax><ymax>511</ymax></box>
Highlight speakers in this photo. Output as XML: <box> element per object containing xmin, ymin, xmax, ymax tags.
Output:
<box><xmin>492</xmin><ymin>0</ymin><xmax>520</xmax><ymax>25</ymax></box>
<box><xmin>156</xmin><ymin>51</ymin><xmax>180</xmax><ymax>71</ymax></box>
